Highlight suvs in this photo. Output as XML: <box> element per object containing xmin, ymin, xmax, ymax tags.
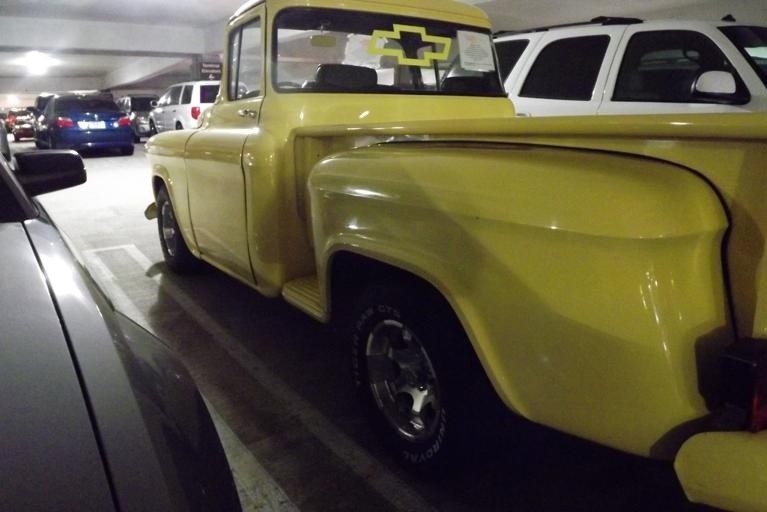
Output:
<box><xmin>148</xmin><ymin>80</ymin><xmax>250</xmax><ymax>137</ymax></box>
<box><xmin>436</xmin><ymin>20</ymin><xmax>766</xmax><ymax>116</ymax></box>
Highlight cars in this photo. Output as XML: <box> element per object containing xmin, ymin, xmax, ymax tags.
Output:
<box><xmin>0</xmin><ymin>125</ymin><xmax>242</xmax><ymax>512</ymax></box>
<box><xmin>7</xmin><ymin>90</ymin><xmax>135</xmax><ymax>154</ymax></box>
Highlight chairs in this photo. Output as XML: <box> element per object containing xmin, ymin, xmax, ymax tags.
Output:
<box><xmin>441</xmin><ymin>76</ymin><xmax>493</xmax><ymax>94</ymax></box>
<box><xmin>302</xmin><ymin>64</ymin><xmax>377</xmax><ymax>90</ymax></box>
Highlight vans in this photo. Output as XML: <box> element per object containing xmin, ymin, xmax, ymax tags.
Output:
<box><xmin>117</xmin><ymin>93</ymin><xmax>159</xmax><ymax>142</ymax></box>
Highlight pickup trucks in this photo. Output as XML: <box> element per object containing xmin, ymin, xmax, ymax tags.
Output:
<box><xmin>145</xmin><ymin>1</ymin><xmax>766</xmax><ymax>512</ymax></box>
